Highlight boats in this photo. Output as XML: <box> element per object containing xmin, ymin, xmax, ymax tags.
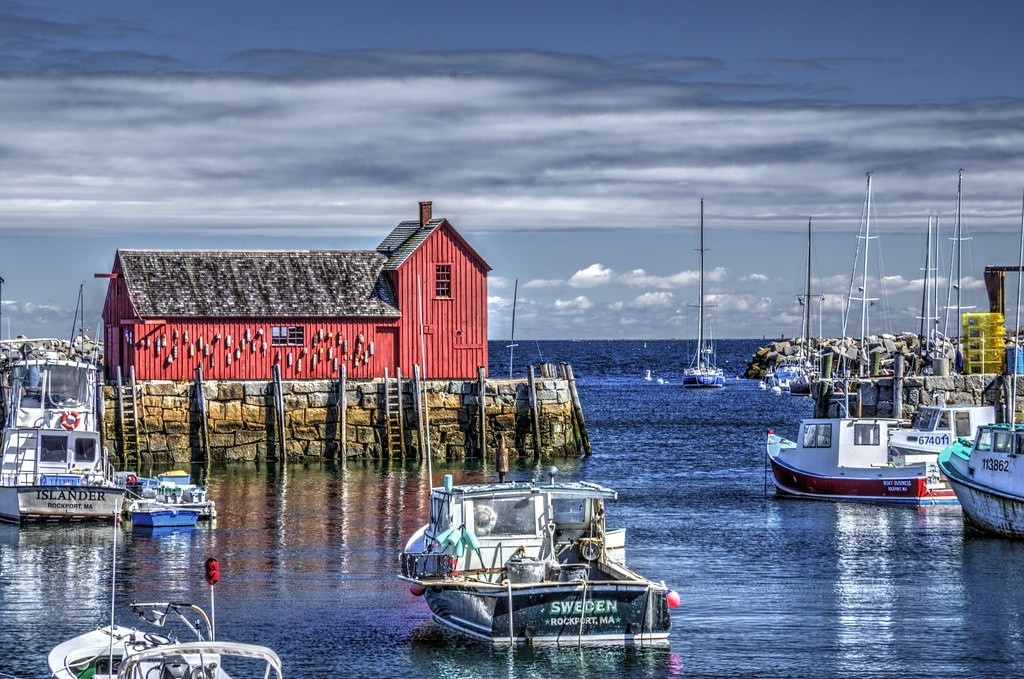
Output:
<box><xmin>47</xmin><ymin>498</ymin><xmax>282</xmax><ymax>678</ymax></box>
<box><xmin>768</xmin><ymin>211</ymin><xmax>1024</xmax><ymax>536</ymax></box>
<box><xmin>397</xmin><ymin>433</ymin><xmax>671</xmax><ymax>646</ymax></box>
<box><xmin>0</xmin><ymin>283</ymin><xmax>216</xmax><ymax>526</ymax></box>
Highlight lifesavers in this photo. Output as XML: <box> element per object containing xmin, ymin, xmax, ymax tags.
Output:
<box><xmin>61</xmin><ymin>409</ymin><xmax>81</xmax><ymax>429</ymax></box>
<box><xmin>473</xmin><ymin>504</ymin><xmax>498</xmax><ymax>536</ymax></box>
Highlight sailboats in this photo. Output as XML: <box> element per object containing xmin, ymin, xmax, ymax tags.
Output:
<box><xmin>765</xmin><ymin>166</ymin><xmax>978</xmax><ymax>392</ymax></box>
<box><xmin>683</xmin><ymin>196</ymin><xmax>726</xmax><ymax>386</ymax></box>
